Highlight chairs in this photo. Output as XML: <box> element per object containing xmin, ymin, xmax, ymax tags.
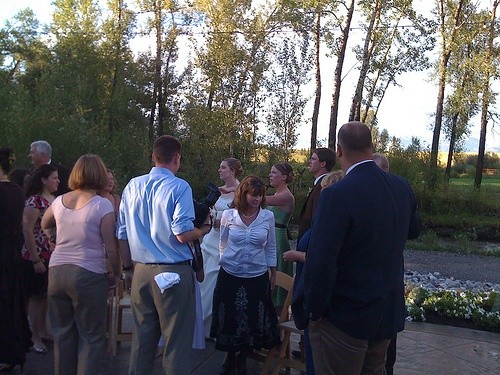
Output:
<box><xmin>105</xmin><ymin>247</ymin><xmax>134</xmax><ymax>357</ymax></box>
<box><xmin>272</xmin><ymin>319</ymin><xmax>307</xmax><ymax>375</ymax></box>
<box><xmin>222</xmin><ymin>268</ymin><xmax>296</xmax><ymax>375</ymax></box>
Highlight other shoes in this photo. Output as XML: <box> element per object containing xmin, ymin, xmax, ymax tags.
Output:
<box><xmin>39</xmin><ymin>331</ymin><xmax>54</xmax><ymax>342</ymax></box>
<box><xmin>236</xmin><ymin>354</ymin><xmax>247</xmax><ymax>375</ymax></box>
<box><xmin>32</xmin><ymin>342</ymin><xmax>47</xmax><ymax>353</ymax></box>
<box><xmin>219</xmin><ymin>355</ymin><xmax>236</xmax><ymax>375</ymax></box>
<box><xmin>292</xmin><ymin>350</ymin><xmax>301</xmax><ymax>358</ymax></box>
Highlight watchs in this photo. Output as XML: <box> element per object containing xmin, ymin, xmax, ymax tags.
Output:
<box><xmin>49</xmin><ymin>235</ymin><xmax>56</xmax><ymax>242</ymax></box>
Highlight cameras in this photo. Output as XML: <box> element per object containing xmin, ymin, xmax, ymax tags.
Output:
<box><xmin>193</xmin><ymin>183</ymin><xmax>222</xmax><ymax>230</ymax></box>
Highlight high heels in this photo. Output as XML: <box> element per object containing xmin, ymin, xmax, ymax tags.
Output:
<box><xmin>0</xmin><ymin>356</ymin><xmax>26</xmax><ymax>373</ymax></box>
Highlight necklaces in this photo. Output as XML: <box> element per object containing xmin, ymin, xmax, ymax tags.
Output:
<box><xmin>238</xmin><ymin>209</ymin><xmax>258</xmax><ymax>218</ymax></box>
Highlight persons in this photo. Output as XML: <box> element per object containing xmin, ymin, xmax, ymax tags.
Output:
<box><xmin>197</xmin><ymin>157</ymin><xmax>245</xmax><ymax>340</ymax></box>
<box><xmin>292</xmin><ymin>148</ymin><xmax>336</xmax><ymax>358</ymax></box>
<box><xmin>282</xmin><ymin>170</ymin><xmax>346</xmax><ymax>375</ymax></box>
<box><xmin>99</xmin><ymin>170</ymin><xmax>121</xmax><ymax>342</ymax></box>
<box><xmin>290</xmin><ymin>122</ymin><xmax>421</xmax><ymax>375</ymax></box>
<box><xmin>371</xmin><ymin>151</ymin><xmax>398</xmax><ymax>375</ymax></box>
<box><xmin>7</xmin><ymin>141</ymin><xmax>70</xmax><ymax>198</ymax></box>
<box><xmin>115</xmin><ymin>135</ymin><xmax>211</xmax><ymax>375</ymax></box>
<box><xmin>22</xmin><ymin>164</ymin><xmax>60</xmax><ymax>354</ymax></box>
<box><xmin>209</xmin><ymin>173</ymin><xmax>278</xmax><ymax>375</ymax></box>
<box><xmin>41</xmin><ymin>154</ymin><xmax>121</xmax><ymax>375</ymax></box>
<box><xmin>0</xmin><ymin>148</ymin><xmax>33</xmax><ymax>375</ymax></box>
<box><xmin>218</xmin><ymin>161</ymin><xmax>296</xmax><ymax>341</ymax></box>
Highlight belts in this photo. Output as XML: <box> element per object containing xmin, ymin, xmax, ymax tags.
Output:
<box><xmin>138</xmin><ymin>260</ymin><xmax>191</xmax><ymax>265</ymax></box>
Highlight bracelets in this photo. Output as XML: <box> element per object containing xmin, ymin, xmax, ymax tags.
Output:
<box><xmin>33</xmin><ymin>259</ymin><xmax>41</xmax><ymax>265</ymax></box>
<box><xmin>121</xmin><ymin>264</ymin><xmax>133</xmax><ymax>272</ymax></box>
